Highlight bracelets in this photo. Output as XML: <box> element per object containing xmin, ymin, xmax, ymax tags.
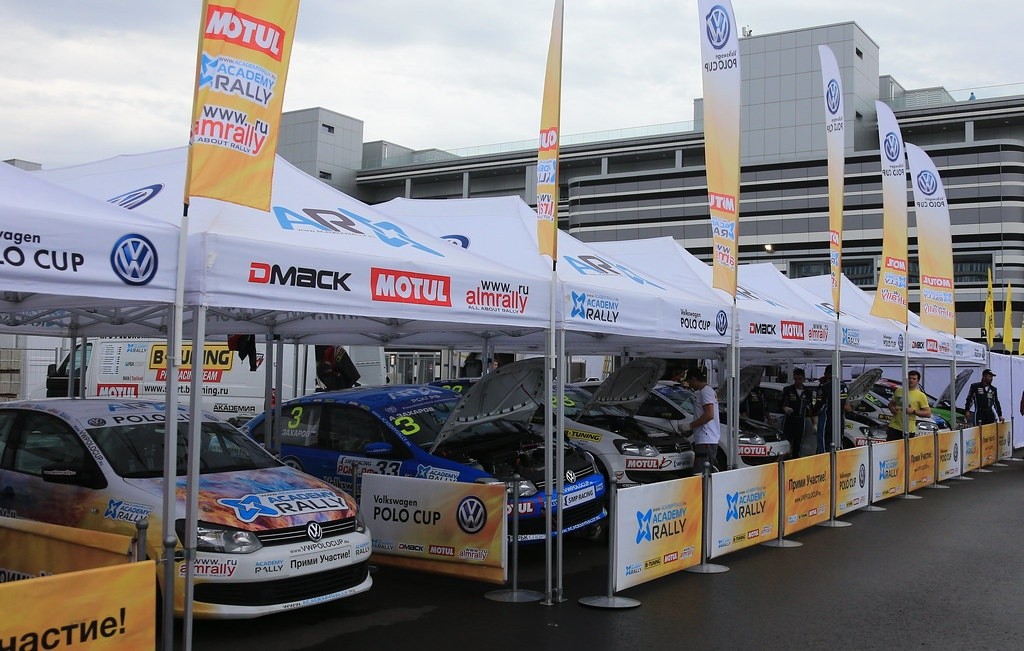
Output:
<box><xmin>913</xmin><ymin>409</ymin><xmax>917</xmax><ymax>414</ymax></box>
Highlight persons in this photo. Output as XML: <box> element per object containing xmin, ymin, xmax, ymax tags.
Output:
<box><xmin>316</xmin><ymin>346</ymin><xmax>345</xmax><ymax>391</ymax></box>
<box><xmin>481</xmin><ymin>358</ymin><xmax>498</xmax><ymax>378</ymax></box>
<box><xmin>781</xmin><ymin>368</ymin><xmax>816</xmax><ymax>459</ymax></box>
<box><xmin>969</xmin><ymin>92</ymin><xmax>975</xmax><ymax>100</ymax></box>
<box><xmin>743</xmin><ymin>378</ymin><xmax>774</xmax><ymax>431</ymax></box>
<box><xmin>814</xmin><ymin>364</ymin><xmax>848</xmax><ymax>455</ymax></box>
<box><xmin>677</xmin><ymin>365</ymin><xmax>721</xmax><ymax>477</ymax></box>
<box><xmin>964</xmin><ymin>369</ymin><xmax>1005</xmax><ymax>425</ymax></box>
<box><xmin>886</xmin><ymin>371</ymin><xmax>931</xmax><ymax>441</ymax></box>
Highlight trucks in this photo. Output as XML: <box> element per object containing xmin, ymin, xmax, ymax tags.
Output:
<box><xmin>46</xmin><ymin>331</ymin><xmax>386</xmax><ymax>422</ymax></box>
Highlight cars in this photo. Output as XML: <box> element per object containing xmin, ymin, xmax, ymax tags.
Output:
<box><xmin>420</xmin><ymin>356</ymin><xmax>697</xmax><ymax>502</ymax></box>
<box><xmin>578</xmin><ymin>356</ymin><xmax>978</xmax><ymax>475</ymax></box>
<box><xmin>0</xmin><ymin>398</ymin><xmax>375</xmax><ymax>632</ymax></box>
<box><xmin>208</xmin><ymin>354</ymin><xmax>608</xmax><ymax>581</ymax></box>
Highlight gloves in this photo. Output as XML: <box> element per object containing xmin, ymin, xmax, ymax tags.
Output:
<box><xmin>784</xmin><ymin>406</ymin><xmax>794</xmax><ymax>414</ymax></box>
<box><xmin>678</xmin><ymin>422</ymin><xmax>693</xmax><ymax>438</ymax></box>
<box><xmin>812</xmin><ymin>424</ymin><xmax>818</xmax><ymax>433</ymax></box>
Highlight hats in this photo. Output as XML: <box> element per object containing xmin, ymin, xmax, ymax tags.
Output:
<box><xmin>982</xmin><ymin>369</ymin><xmax>996</xmax><ymax>376</ymax></box>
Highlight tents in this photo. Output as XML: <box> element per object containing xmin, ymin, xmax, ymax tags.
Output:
<box><xmin>0</xmin><ymin>146</ymin><xmax>987</xmax><ymax>651</ymax></box>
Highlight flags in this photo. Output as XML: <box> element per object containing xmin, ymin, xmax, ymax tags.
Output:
<box><xmin>903</xmin><ymin>141</ymin><xmax>956</xmax><ymax>336</ymax></box>
<box><xmin>869</xmin><ymin>98</ymin><xmax>907</xmax><ymax>323</ymax></box>
<box><xmin>537</xmin><ymin>0</ymin><xmax>565</xmax><ymax>261</ymax></box>
<box><xmin>1003</xmin><ymin>282</ymin><xmax>1014</xmax><ymax>352</ymax></box>
<box><xmin>818</xmin><ymin>42</ymin><xmax>841</xmax><ymax>315</ymax></box>
<box><xmin>1019</xmin><ymin>318</ymin><xmax>1024</xmax><ymax>356</ymax></box>
<box><xmin>984</xmin><ymin>268</ymin><xmax>996</xmax><ymax>347</ymax></box>
<box><xmin>188</xmin><ymin>0</ymin><xmax>299</xmax><ymax>214</ymax></box>
<box><xmin>695</xmin><ymin>0</ymin><xmax>738</xmax><ymax>298</ymax></box>
